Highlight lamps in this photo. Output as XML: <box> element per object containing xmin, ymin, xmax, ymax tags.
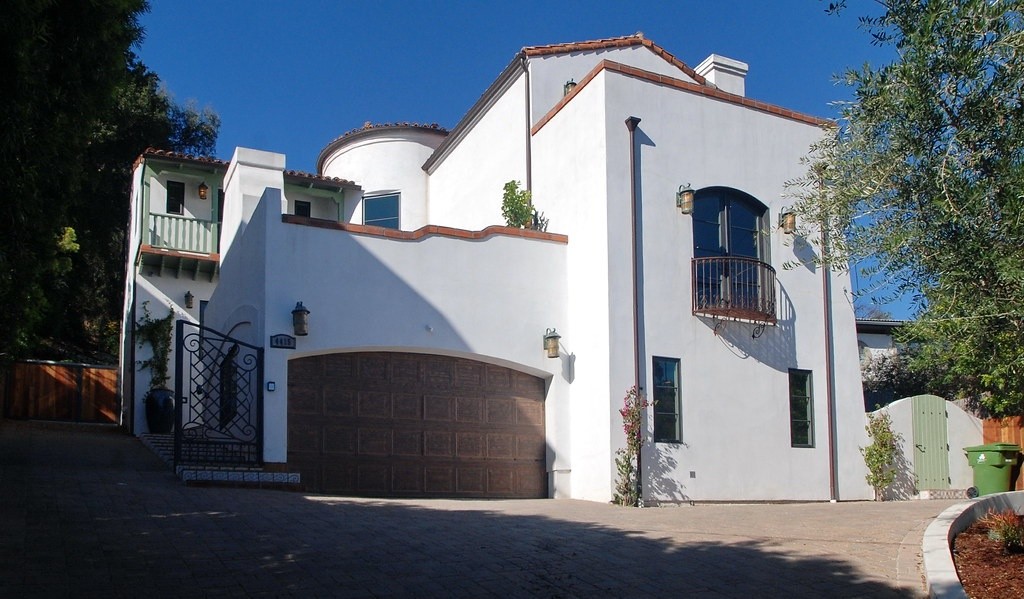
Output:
<box><xmin>525</xmin><ymin>213</ymin><xmax>538</xmax><ymax>229</ymax></box>
<box><xmin>779</xmin><ymin>206</ymin><xmax>797</xmax><ymax>234</ymax></box>
<box><xmin>544</xmin><ymin>327</ymin><xmax>561</xmax><ymax>359</ymax></box>
<box><xmin>185</xmin><ymin>291</ymin><xmax>194</xmax><ymax>308</ymax></box>
<box><xmin>676</xmin><ymin>183</ymin><xmax>694</xmax><ymax>215</ymax></box>
<box><xmin>291</xmin><ymin>301</ymin><xmax>309</xmax><ymax>336</ymax></box>
<box><xmin>564</xmin><ymin>78</ymin><xmax>577</xmax><ymax>95</ymax></box>
<box><xmin>198</xmin><ymin>182</ymin><xmax>208</xmax><ymax>199</ymax></box>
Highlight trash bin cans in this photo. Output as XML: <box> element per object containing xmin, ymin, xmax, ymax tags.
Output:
<box><xmin>962</xmin><ymin>441</ymin><xmax>1022</xmax><ymax>499</ymax></box>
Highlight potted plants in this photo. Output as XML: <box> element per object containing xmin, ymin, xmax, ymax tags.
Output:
<box><xmin>132</xmin><ymin>301</ymin><xmax>178</xmax><ymax>432</ymax></box>
